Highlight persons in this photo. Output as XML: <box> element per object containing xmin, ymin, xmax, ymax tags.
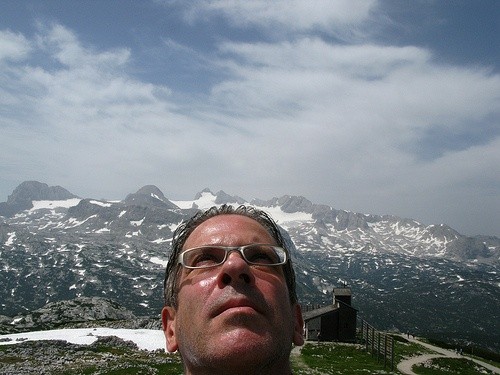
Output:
<box><xmin>159</xmin><ymin>201</ymin><xmax>305</xmax><ymax>374</ymax></box>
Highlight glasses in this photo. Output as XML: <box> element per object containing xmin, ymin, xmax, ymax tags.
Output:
<box><xmin>177</xmin><ymin>243</ymin><xmax>288</xmax><ymax>269</ymax></box>
